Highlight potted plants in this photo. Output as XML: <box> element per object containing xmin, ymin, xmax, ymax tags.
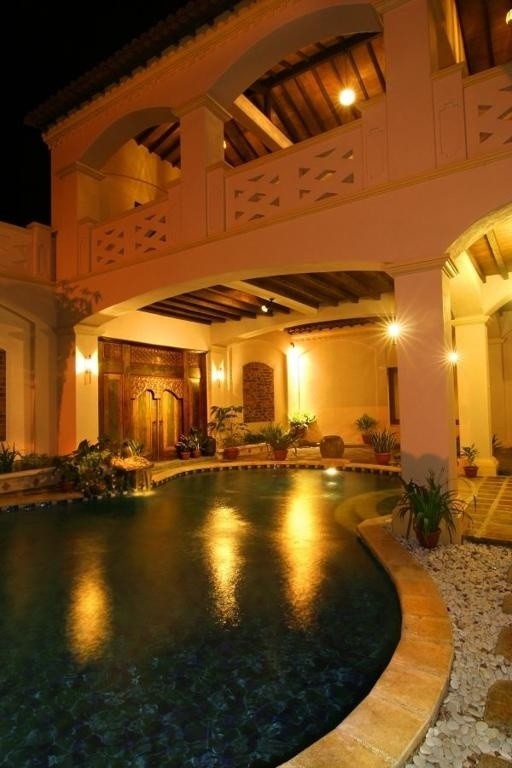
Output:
<box><xmin>388</xmin><ymin>462</ymin><xmax>479</xmax><ymax>553</ymax></box>
<box><xmin>175</xmin><ymin>420</ymin><xmax>306</xmax><ymax>461</ymax></box>
<box><xmin>52</xmin><ymin>439</ymin><xmax>114</xmax><ymax>495</ymax></box>
<box><xmin>457</xmin><ymin>442</ymin><xmax>479</xmax><ymax>478</ymax></box>
<box><xmin>353</xmin><ymin>413</ymin><xmax>398</xmax><ymax>464</ymax></box>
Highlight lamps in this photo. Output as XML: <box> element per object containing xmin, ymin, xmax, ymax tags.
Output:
<box><xmin>261</xmin><ymin>297</ymin><xmax>274</xmax><ymax>313</ymax></box>
